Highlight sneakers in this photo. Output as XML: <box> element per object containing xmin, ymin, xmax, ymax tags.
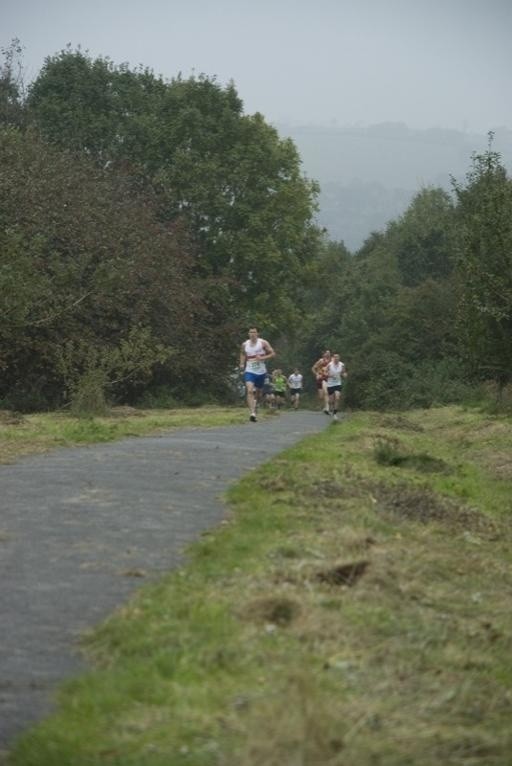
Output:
<box><xmin>323</xmin><ymin>407</ymin><xmax>339</xmax><ymax>421</ymax></box>
<box><xmin>249</xmin><ymin>413</ymin><xmax>257</xmax><ymax>421</ymax></box>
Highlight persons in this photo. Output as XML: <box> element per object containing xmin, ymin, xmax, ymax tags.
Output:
<box><xmin>288</xmin><ymin>366</ymin><xmax>304</xmax><ymax>410</ymax></box>
<box><xmin>262</xmin><ymin>369</ymin><xmax>276</xmax><ymax>408</ymax></box>
<box><xmin>272</xmin><ymin>367</ymin><xmax>289</xmax><ymax>413</ymax></box>
<box><xmin>323</xmin><ymin>350</ymin><xmax>349</xmax><ymax>420</ymax></box>
<box><xmin>239</xmin><ymin>324</ymin><xmax>276</xmax><ymax>423</ymax></box>
<box><xmin>311</xmin><ymin>348</ymin><xmax>332</xmax><ymax>415</ymax></box>
